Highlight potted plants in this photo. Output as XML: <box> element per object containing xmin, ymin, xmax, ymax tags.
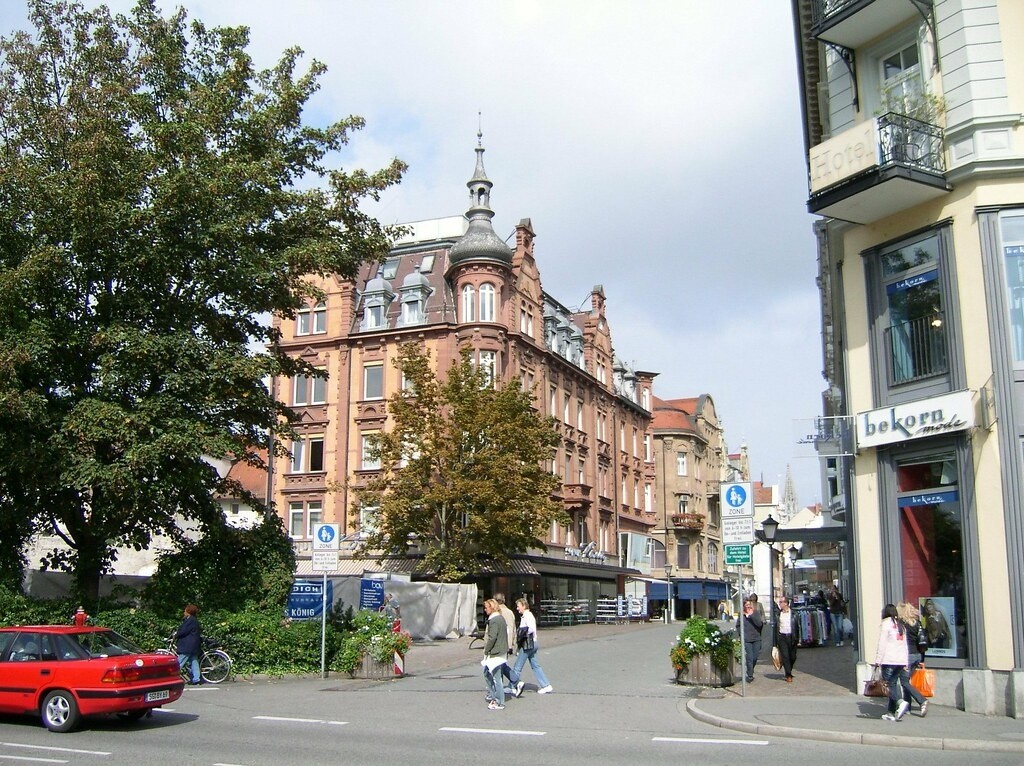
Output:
<box><xmin>864</xmin><ymin>72</ymin><xmax>949</xmax><ymax>164</ymax></box>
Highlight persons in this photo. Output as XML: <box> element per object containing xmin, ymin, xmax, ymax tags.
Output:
<box><xmin>896</xmin><ymin>602</ymin><xmax>929</xmax><ymax>717</ymax></box>
<box><xmin>750</xmin><ymin>593</ymin><xmax>768</xmax><ymax>623</ymax></box>
<box><xmin>794</xmin><ymin>595</ymin><xmax>799</xmax><ymax>602</ymax></box>
<box><xmin>877</xmin><ymin>604</ymin><xmax>909</xmax><ymax>721</ymax></box>
<box><xmin>481</xmin><ymin>598</ymin><xmax>510</xmax><ymax>709</ymax></box>
<box><xmin>483</xmin><ymin>593</ymin><xmax>525</xmax><ymax>700</ymax></box>
<box><xmin>9</xmin><ymin>635</ymin><xmax>35</xmax><ymax>660</ymax></box>
<box><xmin>736</xmin><ymin>600</ymin><xmax>762</xmax><ymax>682</ymax></box>
<box><xmin>172</xmin><ymin>604</ymin><xmax>203</xmax><ymax>685</ymax></box>
<box><xmin>718</xmin><ymin>600</ymin><xmax>728</xmax><ymax>615</ymax></box>
<box><xmin>831</xmin><ymin>585</ymin><xmax>839</xmax><ymax>592</ymax></box>
<box><xmin>802</xmin><ymin>585</ymin><xmax>810</xmax><ymax>595</ymax></box>
<box><xmin>924</xmin><ymin>598</ymin><xmax>951</xmax><ymax>648</ymax></box>
<box><xmin>503</xmin><ymin>598</ymin><xmax>553</xmax><ymax>693</ymax></box>
<box><xmin>776</xmin><ymin>597</ymin><xmax>800</xmax><ymax>682</ymax></box>
<box><xmin>829</xmin><ymin>591</ymin><xmax>846</xmax><ymax>646</ymax></box>
<box><xmin>812</xmin><ymin>590</ymin><xmax>828</xmax><ymax>609</ymax></box>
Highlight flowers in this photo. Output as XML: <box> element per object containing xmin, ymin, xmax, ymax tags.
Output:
<box><xmin>669</xmin><ymin>615</ymin><xmax>731</xmax><ymax>670</ymax></box>
<box><xmin>335</xmin><ymin>607</ymin><xmax>413</xmax><ymax>673</ymax></box>
<box><xmin>673</xmin><ymin>512</ymin><xmax>706</xmax><ymax>529</ymax></box>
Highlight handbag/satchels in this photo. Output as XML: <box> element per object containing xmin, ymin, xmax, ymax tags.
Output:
<box><xmin>843</xmin><ymin>616</ymin><xmax>853</xmax><ymax>633</ymax></box>
<box><xmin>863</xmin><ymin>666</ymin><xmax>890</xmax><ymax>697</ymax></box>
<box><xmin>911</xmin><ymin>665</ymin><xmax>935</xmax><ymax>697</ymax></box>
<box><xmin>772</xmin><ymin>646</ymin><xmax>783</xmax><ymax>671</ymax></box>
<box><xmin>918</xmin><ymin>620</ymin><xmax>928</xmax><ymax>653</ymax></box>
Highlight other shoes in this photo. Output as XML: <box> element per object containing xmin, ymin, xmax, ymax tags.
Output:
<box><xmin>836</xmin><ymin>640</ymin><xmax>844</xmax><ymax>646</ymax></box>
<box><xmin>882</xmin><ymin>714</ymin><xmax>896</xmax><ymax>721</ymax></box>
<box><xmin>748</xmin><ymin>676</ymin><xmax>753</xmax><ymax>681</ymax></box>
<box><xmin>187</xmin><ymin>680</ymin><xmax>202</xmax><ymax>685</ymax></box>
<box><xmin>786</xmin><ymin>674</ymin><xmax>792</xmax><ymax>682</ymax></box>
<box><xmin>485</xmin><ymin>694</ymin><xmax>494</xmax><ymax>702</ymax></box>
<box><xmin>920</xmin><ymin>700</ymin><xmax>929</xmax><ymax>717</ymax></box>
<box><xmin>906</xmin><ymin>710</ymin><xmax>910</xmax><ymax>714</ymax></box>
<box><xmin>488</xmin><ymin>700</ymin><xmax>505</xmax><ymax>709</ymax></box>
<box><xmin>896</xmin><ymin>700</ymin><xmax>909</xmax><ymax>718</ymax></box>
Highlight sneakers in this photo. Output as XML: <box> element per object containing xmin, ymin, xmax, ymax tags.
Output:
<box><xmin>515</xmin><ymin>681</ymin><xmax>525</xmax><ymax>697</ymax></box>
<box><xmin>538</xmin><ymin>685</ymin><xmax>553</xmax><ymax>694</ymax></box>
<box><xmin>503</xmin><ymin>686</ymin><xmax>516</xmax><ymax>694</ymax></box>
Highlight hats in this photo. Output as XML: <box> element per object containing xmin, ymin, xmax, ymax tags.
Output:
<box><xmin>186</xmin><ymin>605</ymin><xmax>198</xmax><ymax>614</ymax></box>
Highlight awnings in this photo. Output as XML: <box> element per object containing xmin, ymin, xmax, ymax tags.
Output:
<box><xmin>630</xmin><ymin>576</ymin><xmax>673</xmax><ymax>584</ymax></box>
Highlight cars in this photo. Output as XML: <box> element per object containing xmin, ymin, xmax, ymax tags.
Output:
<box><xmin>0</xmin><ymin>624</ymin><xmax>186</xmax><ymax>734</ymax></box>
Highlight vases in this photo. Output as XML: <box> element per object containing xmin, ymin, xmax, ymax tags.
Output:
<box><xmin>676</xmin><ymin>653</ymin><xmax>734</xmax><ymax>686</ymax></box>
<box><xmin>350</xmin><ymin>653</ymin><xmax>407</xmax><ymax>680</ymax></box>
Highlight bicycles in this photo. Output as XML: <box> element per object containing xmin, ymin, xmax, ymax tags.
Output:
<box><xmin>154</xmin><ymin>629</ymin><xmax>232</xmax><ymax>684</ymax></box>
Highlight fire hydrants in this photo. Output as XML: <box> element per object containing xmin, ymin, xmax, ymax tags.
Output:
<box><xmin>70</xmin><ymin>605</ymin><xmax>90</xmax><ymax>626</ymax></box>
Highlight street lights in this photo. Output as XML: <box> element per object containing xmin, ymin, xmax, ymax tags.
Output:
<box><xmin>761</xmin><ymin>514</ymin><xmax>779</xmax><ymax>627</ymax></box>
<box><xmin>788</xmin><ymin>544</ymin><xmax>799</xmax><ymax>608</ymax></box>
